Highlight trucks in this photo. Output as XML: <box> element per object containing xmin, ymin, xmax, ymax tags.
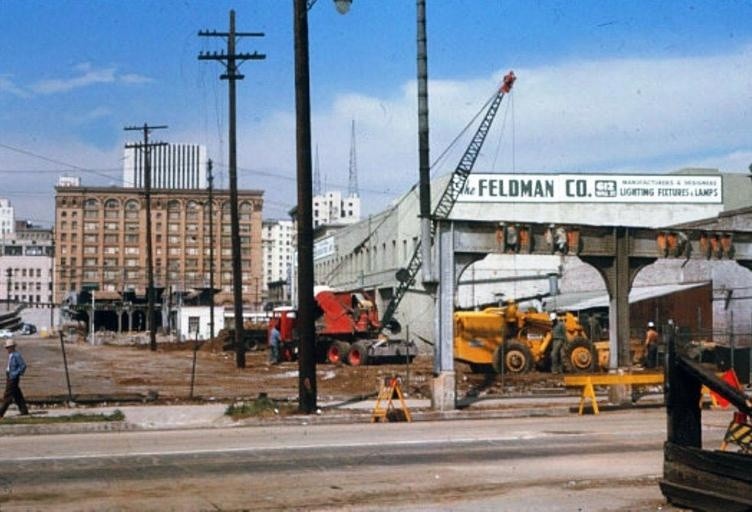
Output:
<box><xmin>224</xmin><ymin>306</ymin><xmax>297</xmax><ymax>351</ymax></box>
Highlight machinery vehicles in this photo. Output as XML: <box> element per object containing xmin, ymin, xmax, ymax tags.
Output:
<box><xmin>452</xmin><ymin>304</ymin><xmax>598</xmax><ymax>377</ymax></box>
<box><xmin>317</xmin><ymin>70</ymin><xmax>517</xmax><ymax>366</ymax></box>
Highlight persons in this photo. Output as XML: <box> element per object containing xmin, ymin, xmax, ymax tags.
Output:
<box><xmin>268</xmin><ymin>324</ymin><xmax>282</xmax><ymax>364</ymax></box>
<box><xmin>549</xmin><ymin>312</ymin><xmax>566</xmax><ymax>374</ymax></box>
<box><xmin>0</xmin><ymin>339</ymin><xmax>29</xmax><ymax>417</ymax></box>
<box><xmin>532</xmin><ymin>293</ymin><xmax>544</xmax><ymax>313</ymax></box>
<box><xmin>645</xmin><ymin>319</ymin><xmax>675</xmax><ymax>369</ymax></box>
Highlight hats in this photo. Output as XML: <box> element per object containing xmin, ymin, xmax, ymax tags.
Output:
<box><xmin>4</xmin><ymin>339</ymin><xmax>15</xmax><ymax>348</ymax></box>
